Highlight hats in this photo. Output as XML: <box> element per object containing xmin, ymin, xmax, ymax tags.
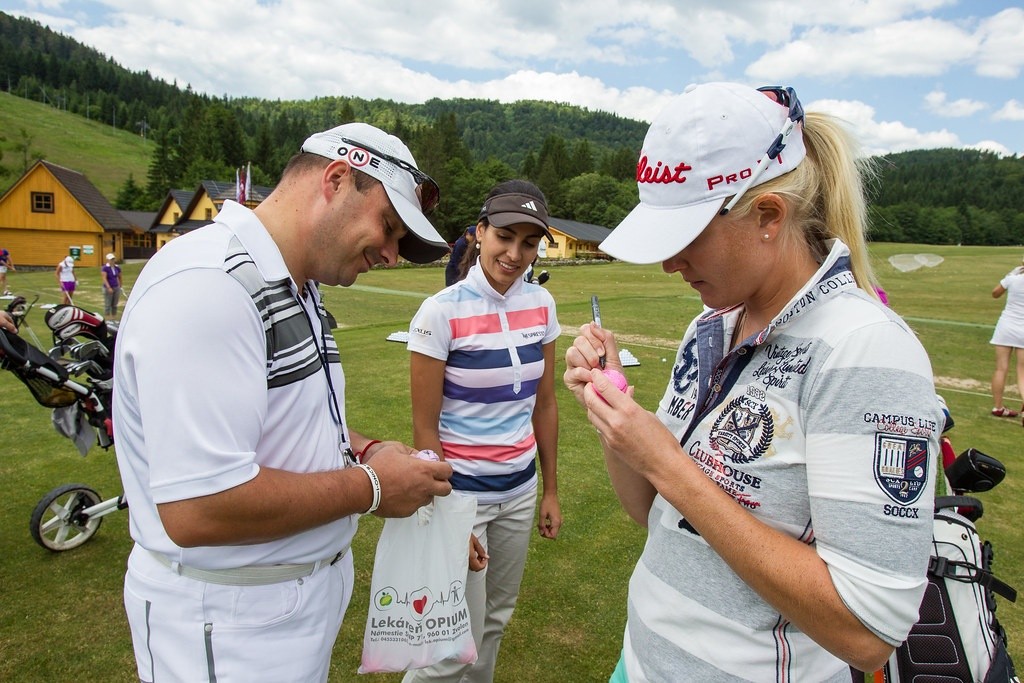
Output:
<box><xmin>106</xmin><ymin>253</ymin><xmax>116</xmax><ymax>260</ymax></box>
<box><xmin>597</xmin><ymin>83</ymin><xmax>807</xmax><ymax>264</ymax></box>
<box><xmin>65</xmin><ymin>256</ymin><xmax>75</xmax><ymax>268</ymax></box>
<box><xmin>479</xmin><ymin>194</ymin><xmax>558</xmax><ymax>245</ymax></box>
<box><xmin>301</xmin><ymin>122</ymin><xmax>450</xmax><ymax>264</ymax></box>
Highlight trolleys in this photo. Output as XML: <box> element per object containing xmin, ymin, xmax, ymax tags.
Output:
<box><xmin>0</xmin><ymin>290</ymin><xmax>130</xmax><ymax>553</ymax></box>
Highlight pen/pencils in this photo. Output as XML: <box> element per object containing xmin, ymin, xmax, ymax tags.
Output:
<box><xmin>591</xmin><ymin>296</ymin><xmax>607</xmax><ymax>370</ymax></box>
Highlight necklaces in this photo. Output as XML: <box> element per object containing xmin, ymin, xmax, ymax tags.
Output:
<box><xmin>741</xmin><ymin>313</ymin><xmax>748</xmax><ymax>343</ymax></box>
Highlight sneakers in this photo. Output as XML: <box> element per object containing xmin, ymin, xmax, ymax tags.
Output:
<box><xmin>992</xmin><ymin>407</ymin><xmax>1019</xmax><ymax>417</ymax></box>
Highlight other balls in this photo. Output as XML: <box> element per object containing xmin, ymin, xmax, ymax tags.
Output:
<box><xmin>593</xmin><ymin>368</ymin><xmax>627</xmax><ymax>401</ymax></box>
<box><xmin>416</xmin><ymin>449</ymin><xmax>441</xmax><ymax>463</ymax></box>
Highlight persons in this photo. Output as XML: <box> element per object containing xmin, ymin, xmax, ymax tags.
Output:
<box><xmin>0</xmin><ymin>309</ymin><xmax>18</xmax><ymax>334</ymax></box>
<box><xmin>402</xmin><ymin>181</ymin><xmax>560</xmax><ymax>683</ymax></box>
<box><xmin>112</xmin><ymin>123</ymin><xmax>453</xmax><ymax>683</ymax></box>
<box><xmin>564</xmin><ymin>82</ymin><xmax>946</xmax><ymax>683</ymax></box>
<box><xmin>445</xmin><ymin>227</ymin><xmax>550</xmax><ymax>289</ymax></box>
<box><xmin>0</xmin><ymin>248</ymin><xmax>16</xmax><ymax>294</ymax></box>
<box><xmin>102</xmin><ymin>253</ymin><xmax>122</xmax><ymax>324</ymax></box>
<box><xmin>990</xmin><ymin>260</ymin><xmax>1024</xmax><ymax>427</ymax></box>
<box><xmin>56</xmin><ymin>256</ymin><xmax>80</xmax><ymax>305</ymax></box>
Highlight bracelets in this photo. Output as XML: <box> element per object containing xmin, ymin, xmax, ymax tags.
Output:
<box><xmin>355</xmin><ymin>440</ymin><xmax>381</xmax><ymax>464</ymax></box>
<box><xmin>352</xmin><ymin>464</ymin><xmax>381</xmax><ymax>515</ymax></box>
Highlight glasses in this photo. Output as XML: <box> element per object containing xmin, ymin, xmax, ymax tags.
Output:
<box><xmin>342</xmin><ymin>135</ymin><xmax>441</xmax><ymax>215</ymax></box>
<box><xmin>720</xmin><ymin>85</ymin><xmax>804</xmax><ymax>218</ymax></box>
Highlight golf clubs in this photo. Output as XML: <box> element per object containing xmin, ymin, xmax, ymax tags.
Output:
<box><xmin>63</xmin><ymin>288</ymin><xmax>75</xmax><ymax>306</ymax></box>
<box><xmin>14</xmin><ymin>270</ymin><xmax>34</xmax><ymax>285</ymax></box>
<box><xmin>47</xmin><ymin>334</ymin><xmax>109</xmax><ymax>378</ymax></box>
<box><xmin>119</xmin><ymin>287</ymin><xmax>128</xmax><ymax>299</ymax></box>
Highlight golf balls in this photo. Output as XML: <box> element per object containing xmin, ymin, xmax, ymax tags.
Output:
<box><xmin>386</xmin><ymin>331</ymin><xmax>410</xmax><ymax>342</ymax></box>
<box><xmin>619</xmin><ymin>349</ymin><xmax>640</xmax><ymax>365</ymax></box>
<box><xmin>662</xmin><ymin>358</ymin><xmax>667</xmax><ymax>362</ymax></box>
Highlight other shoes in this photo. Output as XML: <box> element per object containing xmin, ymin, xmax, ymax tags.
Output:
<box><xmin>3</xmin><ymin>291</ymin><xmax>13</xmax><ymax>295</ymax></box>
<box><xmin>106</xmin><ymin>319</ymin><xmax>119</xmax><ymax>325</ymax></box>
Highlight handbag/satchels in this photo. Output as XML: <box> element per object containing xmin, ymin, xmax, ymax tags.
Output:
<box><xmin>357</xmin><ymin>492</ymin><xmax>479</xmax><ymax>675</ymax></box>
